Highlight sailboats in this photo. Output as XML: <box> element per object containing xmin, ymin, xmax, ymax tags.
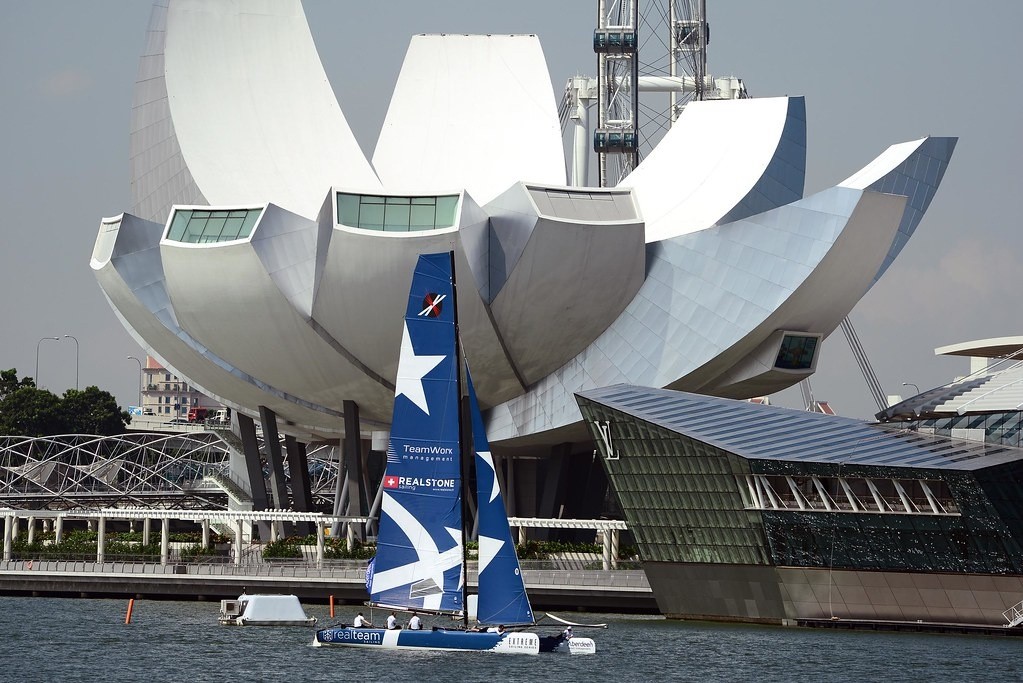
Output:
<box><xmin>304</xmin><ymin>248</ymin><xmax>612</xmax><ymax>657</ymax></box>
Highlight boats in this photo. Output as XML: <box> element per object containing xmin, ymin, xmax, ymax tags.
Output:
<box><xmin>216</xmin><ymin>592</ymin><xmax>319</xmax><ymax>629</ymax></box>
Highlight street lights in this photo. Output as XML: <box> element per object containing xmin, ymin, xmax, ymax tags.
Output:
<box><xmin>903</xmin><ymin>382</ymin><xmax>920</xmax><ymax>429</ymax></box>
<box><xmin>64</xmin><ymin>335</ymin><xmax>80</xmax><ymax>392</ymax></box>
<box><xmin>126</xmin><ymin>356</ymin><xmax>142</xmax><ymax>407</ymax></box>
<box><xmin>35</xmin><ymin>336</ymin><xmax>62</xmax><ymax>389</ymax></box>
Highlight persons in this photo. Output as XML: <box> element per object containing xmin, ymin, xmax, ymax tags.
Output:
<box><xmin>465</xmin><ymin>625</ymin><xmax>509</xmax><ymax>636</ymax></box>
<box><xmin>354</xmin><ymin>613</ymin><xmax>372</xmax><ymax>628</ymax></box>
<box><xmin>385</xmin><ymin>612</ymin><xmax>401</xmax><ymax>630</ymax></box>
<box><xmin>407</xmin><ymin>614</ymin><xmax>423</xmax><ymax>630</ymax></box>
<box><xmin>564</xmin><ymin>626</ymin><xmax>573</xmax><ymax>641</ymax></box>
